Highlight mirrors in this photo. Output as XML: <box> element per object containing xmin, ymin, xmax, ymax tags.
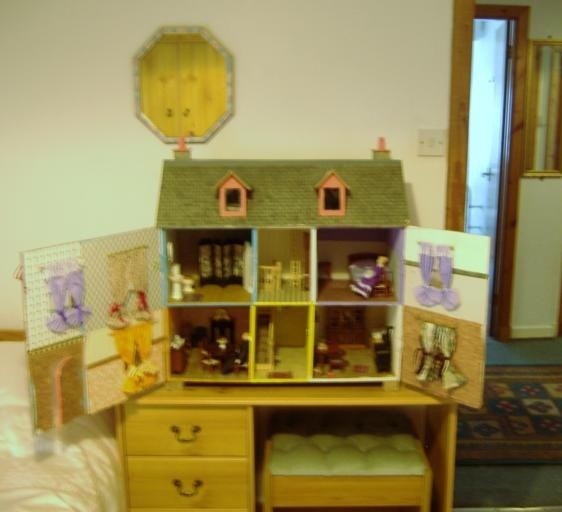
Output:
<box><xmin>130</xmin><ymin>26</ymin><xmax>235</xmax><ymax>145</ymax></box>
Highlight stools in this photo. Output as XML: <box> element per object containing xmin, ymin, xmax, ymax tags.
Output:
<box><xmin>264</xmin><ymin>407</ymin><xmax>432</xmax><ymax>511</ymax></box>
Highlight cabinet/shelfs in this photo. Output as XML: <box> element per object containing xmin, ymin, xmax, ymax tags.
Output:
<box><xmin>115</xmin><ymin>385</ymin><xmax>253</xmax><ymax>512</ymax></box>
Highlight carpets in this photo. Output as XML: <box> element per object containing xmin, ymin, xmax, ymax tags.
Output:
<box><xmin>454</xmin><ymin>363</ymin><xmax>561</xmax><ymax>468</ymax></box>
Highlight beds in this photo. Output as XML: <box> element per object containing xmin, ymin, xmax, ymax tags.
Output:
<box><xmin>2</xmin><ymin>330</ymin><xmax>126</xmax><ymax>511</ymax></box>
